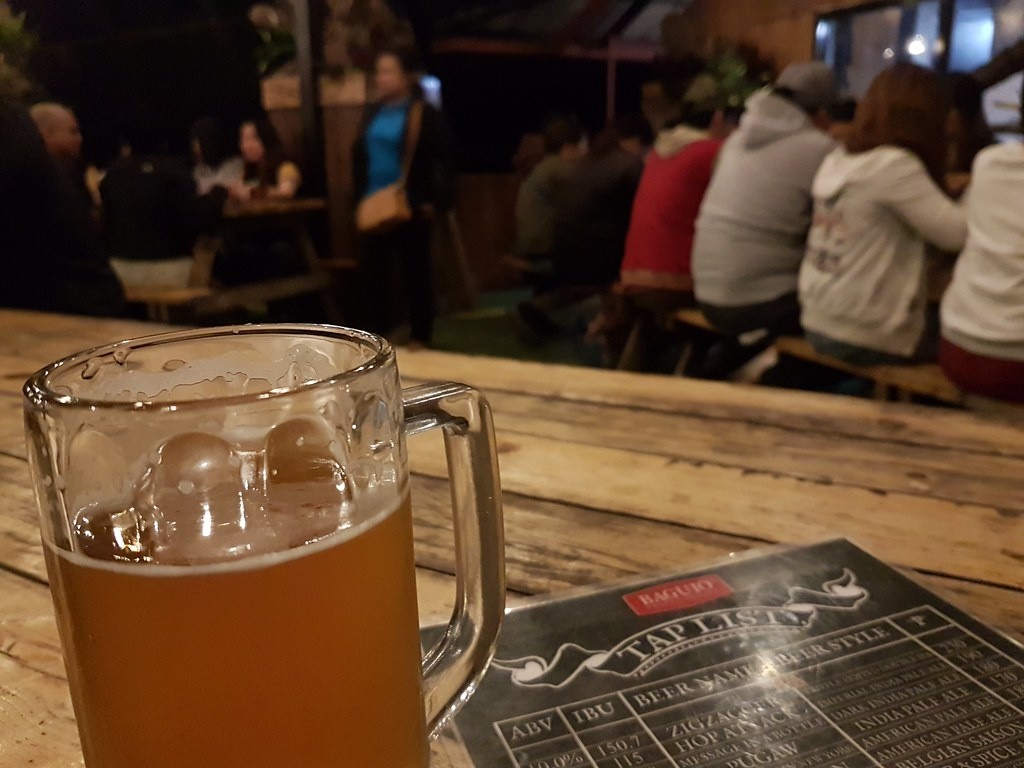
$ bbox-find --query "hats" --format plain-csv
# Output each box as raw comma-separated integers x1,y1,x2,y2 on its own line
776,61,838,112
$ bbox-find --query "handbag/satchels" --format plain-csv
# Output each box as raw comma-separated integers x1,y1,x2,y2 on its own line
356,183,410,232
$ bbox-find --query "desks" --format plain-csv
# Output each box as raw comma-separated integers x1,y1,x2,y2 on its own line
220,200,329,265
0,310,1024,768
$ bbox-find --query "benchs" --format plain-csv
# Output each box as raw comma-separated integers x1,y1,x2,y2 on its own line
615,283,1023,414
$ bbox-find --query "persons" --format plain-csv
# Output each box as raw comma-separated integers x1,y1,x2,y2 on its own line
515,56,1024,401
347,49,450,346
1,98,306,323
